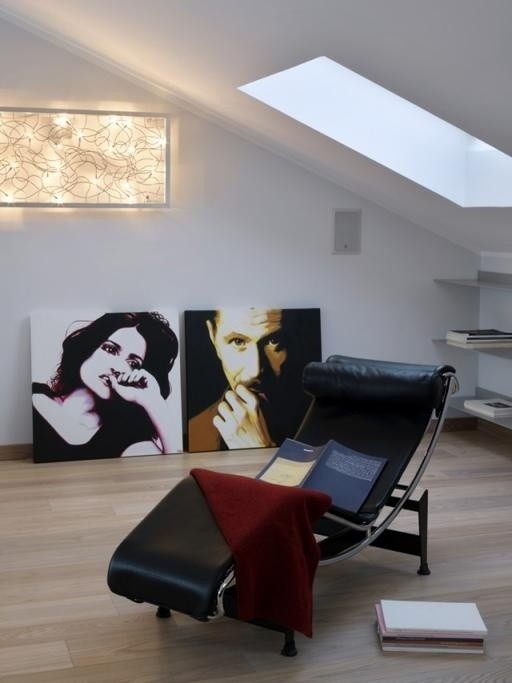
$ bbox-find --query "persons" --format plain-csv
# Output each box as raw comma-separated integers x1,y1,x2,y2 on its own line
187,308,310,450
30,312,184,464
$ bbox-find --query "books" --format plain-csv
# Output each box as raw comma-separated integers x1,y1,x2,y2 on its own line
374,597,487,657
464,395,512,420
445,324,512,346
257,436,390,518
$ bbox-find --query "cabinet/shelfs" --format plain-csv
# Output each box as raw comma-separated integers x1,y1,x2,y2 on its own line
431,279,512,430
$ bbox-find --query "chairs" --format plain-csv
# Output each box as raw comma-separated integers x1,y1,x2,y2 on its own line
108,355,460,657
